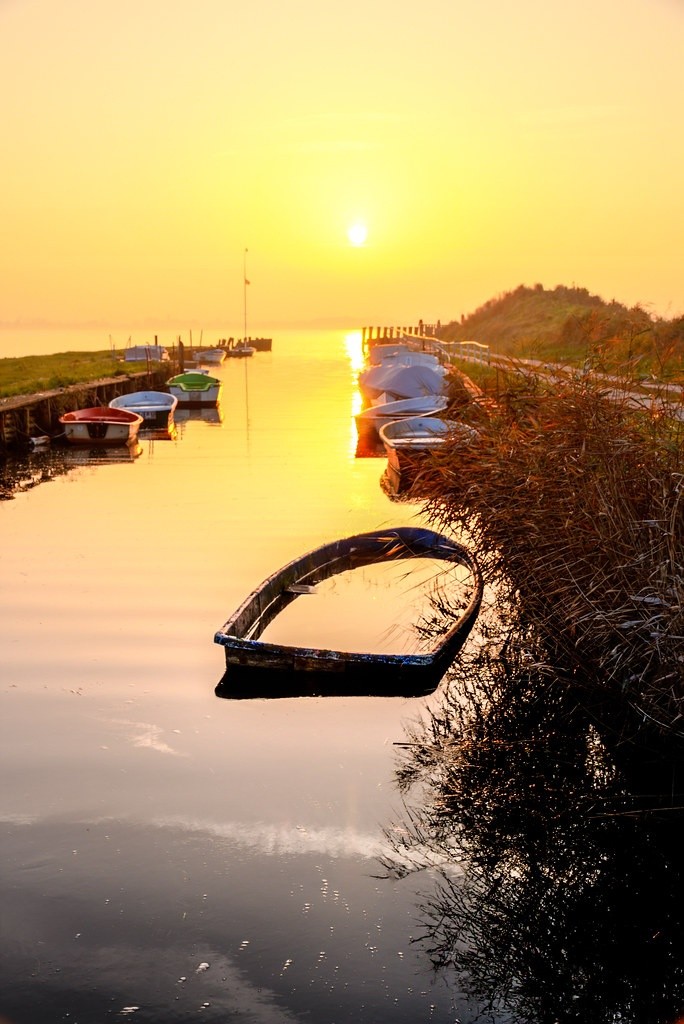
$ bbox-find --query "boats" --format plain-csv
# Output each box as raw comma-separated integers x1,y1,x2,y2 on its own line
352,393,454,432
138,421,183,441
379,414,479,470
165,372,223,405
106,390,179,432
211,526,483,680
196,349,228,364
383,461,418,495
362,338,455,404
173,401,221,423
57,405,144,442
53,443,144,465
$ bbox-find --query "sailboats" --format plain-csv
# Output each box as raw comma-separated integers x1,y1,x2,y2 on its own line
234,247,257,357
